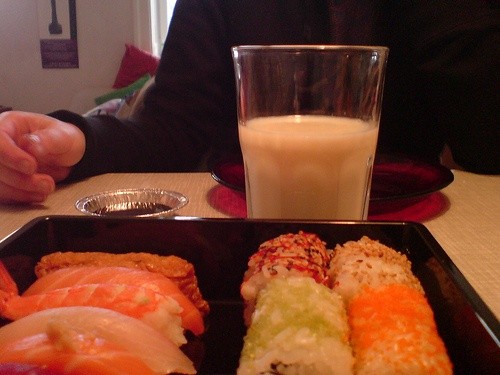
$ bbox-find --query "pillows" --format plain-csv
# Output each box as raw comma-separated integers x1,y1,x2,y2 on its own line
93,42,162,110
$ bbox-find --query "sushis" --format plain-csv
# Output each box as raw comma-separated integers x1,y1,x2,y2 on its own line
233,231,453,375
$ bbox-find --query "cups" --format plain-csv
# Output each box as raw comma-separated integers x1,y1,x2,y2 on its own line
231,44,390,221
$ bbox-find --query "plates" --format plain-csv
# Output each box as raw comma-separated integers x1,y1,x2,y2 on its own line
210,153,456,213
74,188,189,218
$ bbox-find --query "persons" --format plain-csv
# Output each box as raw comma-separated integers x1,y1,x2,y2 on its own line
0,1,500,204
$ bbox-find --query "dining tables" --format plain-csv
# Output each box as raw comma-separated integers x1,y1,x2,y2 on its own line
0,171,500,375
